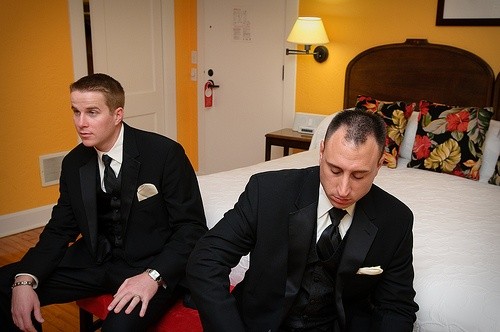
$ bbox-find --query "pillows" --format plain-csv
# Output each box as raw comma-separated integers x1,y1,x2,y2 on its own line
355,94,416,168
407,100,493,181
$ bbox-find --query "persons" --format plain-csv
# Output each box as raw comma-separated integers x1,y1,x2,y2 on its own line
189,107,420,332
0,71,212,332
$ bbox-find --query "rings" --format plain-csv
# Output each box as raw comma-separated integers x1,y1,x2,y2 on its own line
134,295,141,300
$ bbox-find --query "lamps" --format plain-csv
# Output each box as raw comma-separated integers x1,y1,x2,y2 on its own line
285,16,329,63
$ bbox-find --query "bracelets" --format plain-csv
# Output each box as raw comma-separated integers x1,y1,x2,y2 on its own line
10,281,34,288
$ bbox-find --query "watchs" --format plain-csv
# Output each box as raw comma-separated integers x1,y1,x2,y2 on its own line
143,267,163,285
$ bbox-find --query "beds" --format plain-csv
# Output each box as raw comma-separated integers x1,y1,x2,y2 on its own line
76,39,500,332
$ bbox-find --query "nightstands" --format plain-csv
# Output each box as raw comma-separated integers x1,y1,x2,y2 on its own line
265,128,313,162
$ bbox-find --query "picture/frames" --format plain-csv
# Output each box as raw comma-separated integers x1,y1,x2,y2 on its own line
436,0,500,27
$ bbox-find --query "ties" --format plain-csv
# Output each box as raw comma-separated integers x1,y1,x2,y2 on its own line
316,206,348,261
102,155,116,195
